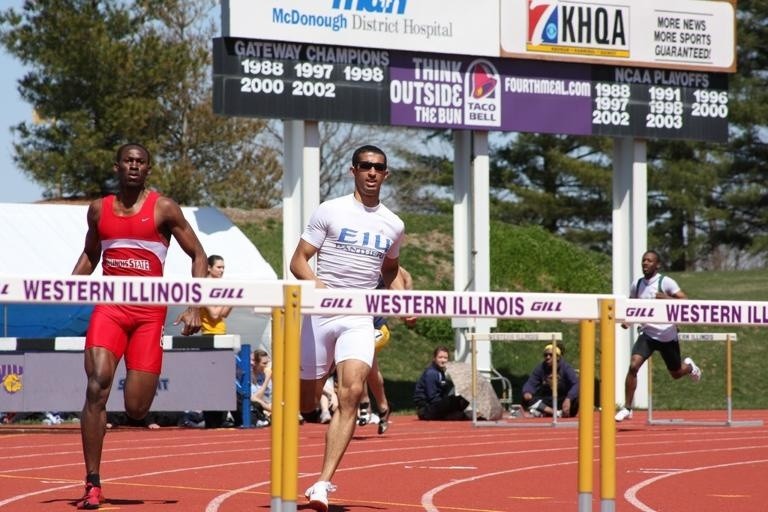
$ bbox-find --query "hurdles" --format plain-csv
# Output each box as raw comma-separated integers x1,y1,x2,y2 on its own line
561,298,768,512
253,288,626,512
640,332,763,426
0,275,315,512
466,332,579,429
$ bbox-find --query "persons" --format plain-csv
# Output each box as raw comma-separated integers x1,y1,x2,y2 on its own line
290,146,417,511
200,255,233,334
71,144,208,509
359,266,417,434
414,346,472,420
614,251,700,422
522,344,578,417
251,350,380,427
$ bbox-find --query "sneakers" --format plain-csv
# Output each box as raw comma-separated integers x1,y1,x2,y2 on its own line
615,408,632,422
304,480,337,512
378,406,391,434
557,410,563,419
77,482,104,509
684,357,701,383
530,409,544,418
320,410,331,424
359,402,371,426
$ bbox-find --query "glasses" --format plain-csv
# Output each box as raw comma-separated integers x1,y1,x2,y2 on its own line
543,352,553,357
353,160,387,172
